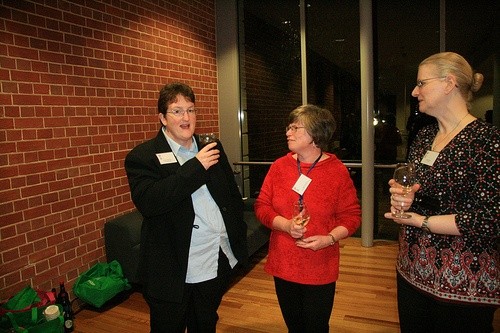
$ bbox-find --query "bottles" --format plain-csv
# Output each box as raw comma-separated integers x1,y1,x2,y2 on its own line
234,171,242,196
57,280,69,305
62,293,73,333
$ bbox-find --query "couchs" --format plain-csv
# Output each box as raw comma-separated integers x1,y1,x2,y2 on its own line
104,199,271,287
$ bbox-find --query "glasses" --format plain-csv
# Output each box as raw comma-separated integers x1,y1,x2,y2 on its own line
417,77,459,88
286,124,306,131
166,107,199,117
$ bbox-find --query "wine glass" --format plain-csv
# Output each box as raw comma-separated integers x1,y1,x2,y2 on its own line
393,164,416,219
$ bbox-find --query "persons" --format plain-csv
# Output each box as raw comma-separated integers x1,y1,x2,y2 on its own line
253,104,363,333
384,52,500,333
125,82,248,333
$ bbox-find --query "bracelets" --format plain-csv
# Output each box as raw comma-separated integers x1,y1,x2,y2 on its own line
328,234,336,244
420,215,430,231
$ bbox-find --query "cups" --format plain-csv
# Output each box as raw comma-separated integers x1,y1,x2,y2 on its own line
294,203,310,228
199,133,216,150
44,305,59,321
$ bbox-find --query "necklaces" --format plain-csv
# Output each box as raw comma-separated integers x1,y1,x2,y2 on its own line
432,112,469,150
